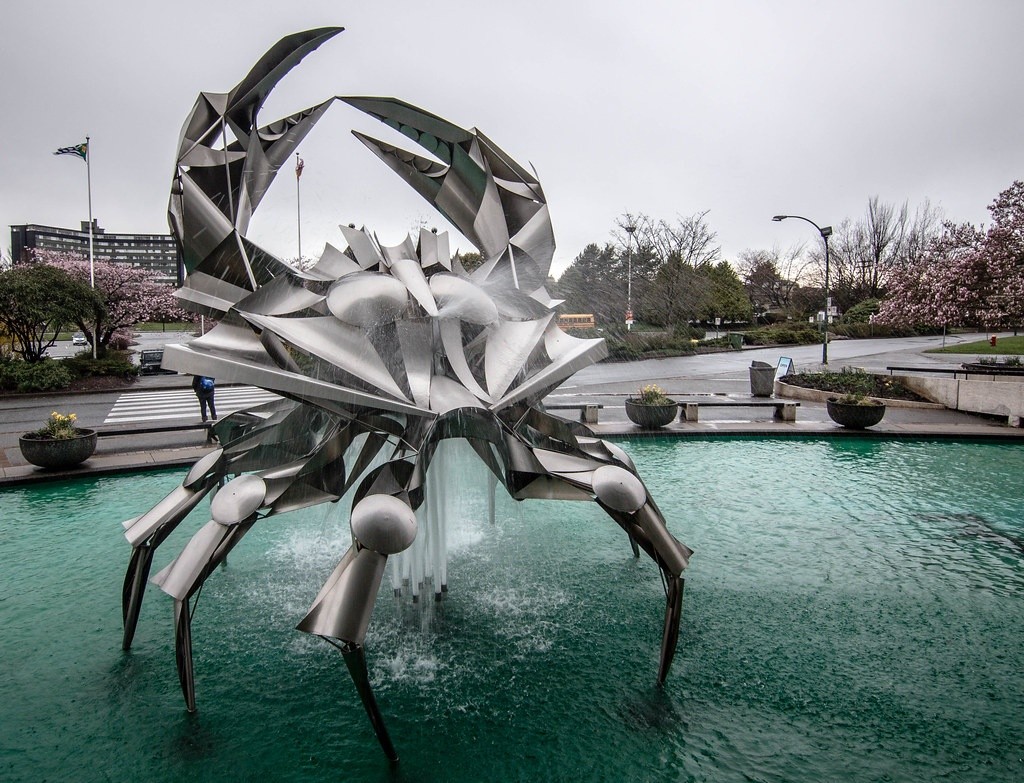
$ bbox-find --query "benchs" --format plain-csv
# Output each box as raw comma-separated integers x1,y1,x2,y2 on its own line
83,421,216,455
544,403,604,424
677,401,801,423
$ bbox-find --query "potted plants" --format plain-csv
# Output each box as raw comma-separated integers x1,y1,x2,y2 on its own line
625,384,678,428
18,412,97,469
826,390,887,429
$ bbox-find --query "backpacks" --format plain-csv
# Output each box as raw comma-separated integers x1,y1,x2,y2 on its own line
200,376,215,393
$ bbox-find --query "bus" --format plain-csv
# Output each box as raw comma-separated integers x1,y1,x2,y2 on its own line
556,314,595,332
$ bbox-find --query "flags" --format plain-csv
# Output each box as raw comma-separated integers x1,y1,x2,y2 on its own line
294,158,304,177
52,142,87,162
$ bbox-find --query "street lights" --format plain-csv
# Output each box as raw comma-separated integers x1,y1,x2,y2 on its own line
625,226,636,331
772,215,830,364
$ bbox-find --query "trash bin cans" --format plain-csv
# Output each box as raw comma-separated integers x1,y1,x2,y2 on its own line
749,360,776,398
729,332,743,345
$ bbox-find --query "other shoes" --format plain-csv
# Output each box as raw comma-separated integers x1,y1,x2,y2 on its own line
202,416,208,422
212,415,217,420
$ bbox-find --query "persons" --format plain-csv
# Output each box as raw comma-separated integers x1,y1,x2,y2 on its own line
190,374,220,424
